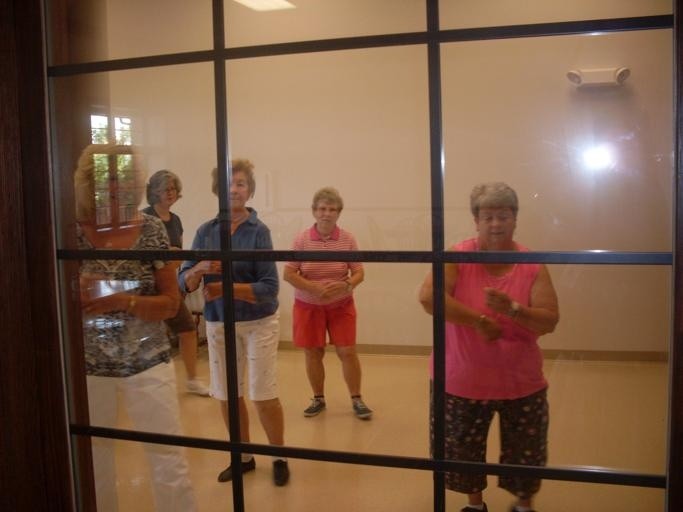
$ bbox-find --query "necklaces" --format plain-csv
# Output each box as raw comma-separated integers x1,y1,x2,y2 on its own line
318,231,331,236
99,235,112,247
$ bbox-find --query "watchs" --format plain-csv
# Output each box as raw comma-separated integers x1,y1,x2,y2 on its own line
510,301,520,322
345,280,353,292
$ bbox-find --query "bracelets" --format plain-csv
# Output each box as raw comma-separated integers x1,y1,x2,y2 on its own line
127,296,135,313
475,315,486,331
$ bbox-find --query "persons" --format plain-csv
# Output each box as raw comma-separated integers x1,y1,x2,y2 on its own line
75,144,196,512
285,188,375,418
179,159,290,486
141,168,211,397
418,183,558,512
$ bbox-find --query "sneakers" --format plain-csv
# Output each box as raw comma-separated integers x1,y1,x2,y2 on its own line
353,400,374,418
186,378,211,396
218,457,256,483
460,501,488,512
272,459,289,486
303,400,326,417
